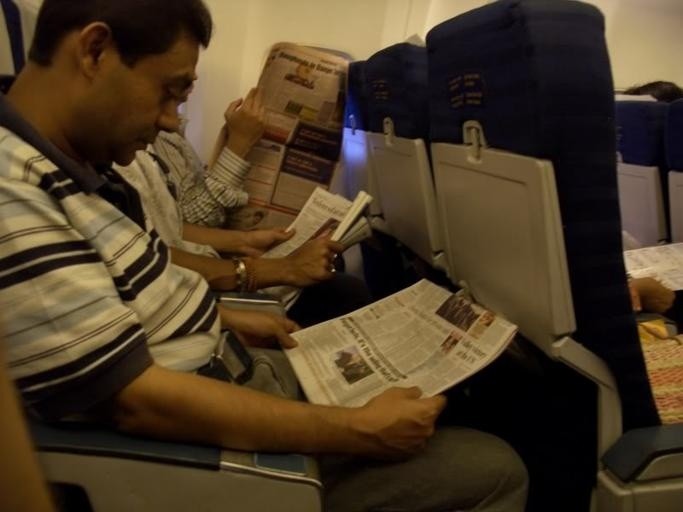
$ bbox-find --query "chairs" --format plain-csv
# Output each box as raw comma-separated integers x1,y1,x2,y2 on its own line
358,42,447,275
615,94,665,245
339,59,387,231
425,0,682,512
0,0,326,512
665,97,682,247
5,0,39,77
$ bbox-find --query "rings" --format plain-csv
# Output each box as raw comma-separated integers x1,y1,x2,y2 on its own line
334,251,342,262
328,263,338,274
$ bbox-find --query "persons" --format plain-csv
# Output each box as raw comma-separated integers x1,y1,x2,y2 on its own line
153,84,271,228
632,275,682,334
241,211,264,228
0,0,530,512
105,147,373,324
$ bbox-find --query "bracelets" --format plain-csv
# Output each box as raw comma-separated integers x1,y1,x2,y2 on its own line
242,255,257,294
233,255,246,294
